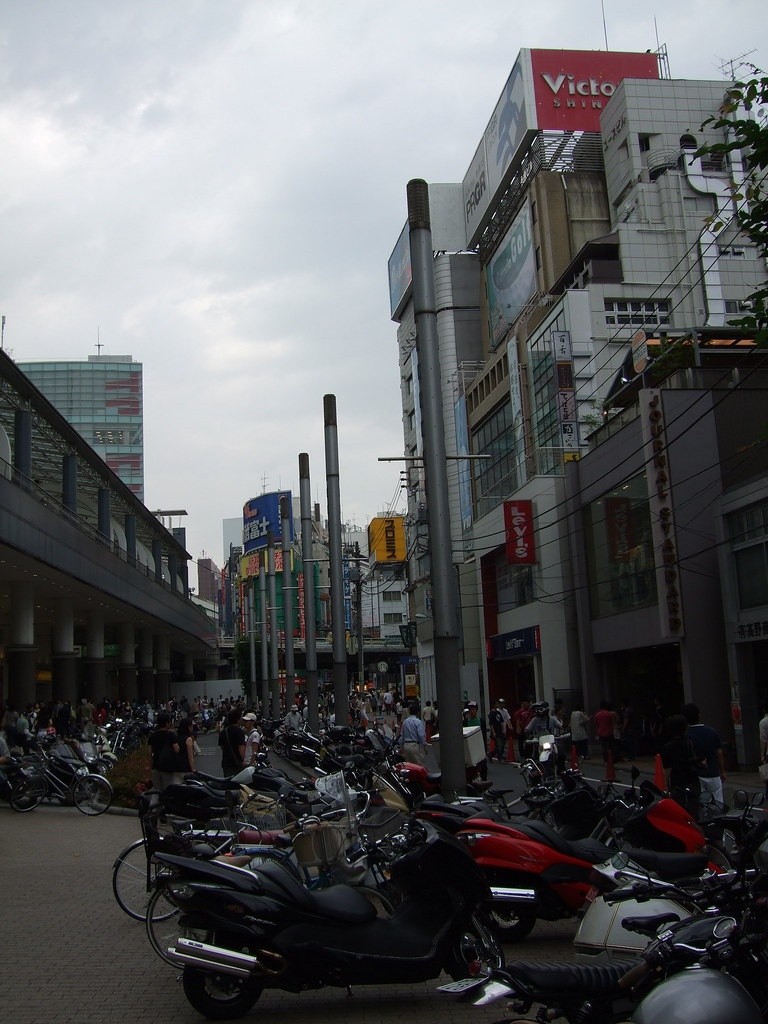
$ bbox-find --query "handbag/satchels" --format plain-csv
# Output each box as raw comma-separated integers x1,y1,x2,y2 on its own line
430,712,435,723
255,748,270,765
232,761,245,774
758,763,768,783
149,729,171,757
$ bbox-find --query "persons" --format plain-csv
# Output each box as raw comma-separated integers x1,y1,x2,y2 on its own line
759,702,768,798
348,679,440,767
269,685,336,737
0,695,263,792
657,703,727,819
462,695,662,781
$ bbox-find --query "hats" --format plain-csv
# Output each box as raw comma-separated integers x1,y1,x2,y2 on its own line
468,701,478,708
290,704,298,711
241,712,257,721
462,709,470,714
498,699,505,703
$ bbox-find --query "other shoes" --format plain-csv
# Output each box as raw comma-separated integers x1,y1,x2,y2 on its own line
487,754,493,764
498,759,506,764
584,756,591,759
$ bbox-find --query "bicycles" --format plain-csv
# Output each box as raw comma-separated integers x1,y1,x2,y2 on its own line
9,742,115,818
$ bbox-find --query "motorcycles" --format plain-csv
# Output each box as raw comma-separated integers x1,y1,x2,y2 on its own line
19,706,160,806
167,702,228,735
257,716,438,806
143,722,768,1023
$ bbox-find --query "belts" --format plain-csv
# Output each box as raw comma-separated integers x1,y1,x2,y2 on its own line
404,740,418,744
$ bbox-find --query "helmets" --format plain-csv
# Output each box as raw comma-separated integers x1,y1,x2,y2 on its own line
534,701,549,717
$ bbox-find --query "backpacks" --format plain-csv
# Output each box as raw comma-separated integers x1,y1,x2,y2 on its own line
166,735,189,772
